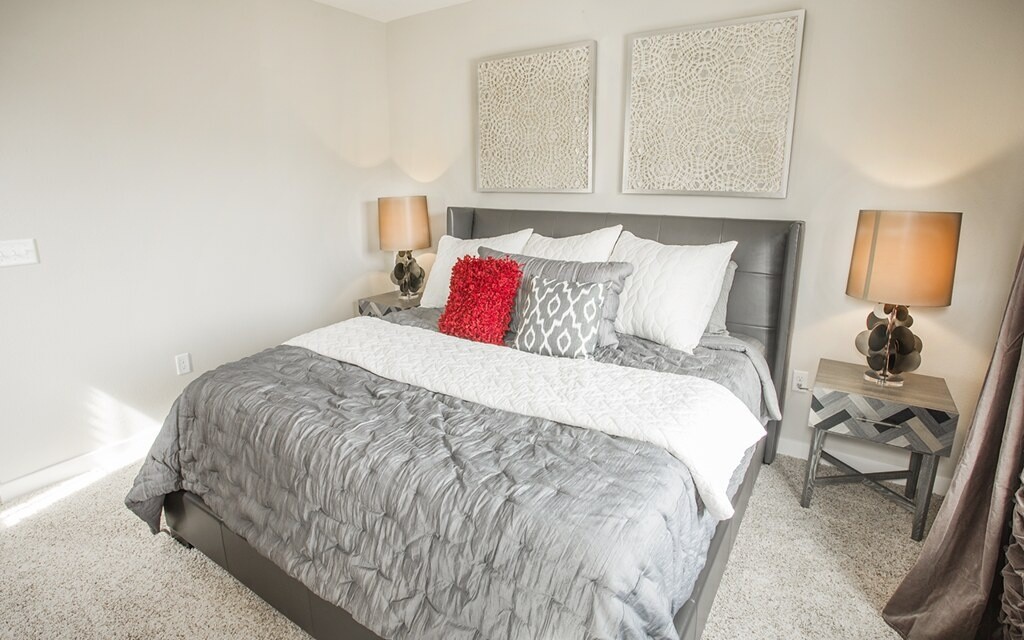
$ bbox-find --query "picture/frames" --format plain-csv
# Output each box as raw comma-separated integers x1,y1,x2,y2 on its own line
475,38,598,194
620,7,806,199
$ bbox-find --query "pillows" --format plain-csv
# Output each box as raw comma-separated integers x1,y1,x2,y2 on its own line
477,244,634,350
522,222,623,263
512,275,614,360
607,231,739,355
420,227,534,309
439,254,526,346
704,260,738,338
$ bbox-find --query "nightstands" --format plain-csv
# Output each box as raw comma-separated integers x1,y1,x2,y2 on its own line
800,357,960,542
358,289,424,317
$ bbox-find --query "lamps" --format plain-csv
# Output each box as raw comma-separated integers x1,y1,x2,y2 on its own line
846,209,962,387
376,195,431,300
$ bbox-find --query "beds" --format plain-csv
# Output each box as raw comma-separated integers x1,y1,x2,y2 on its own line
124,206,808,640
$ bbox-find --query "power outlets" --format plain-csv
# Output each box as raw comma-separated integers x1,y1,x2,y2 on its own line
792,370,809,392
176,352,191,375
0,238,40,266
